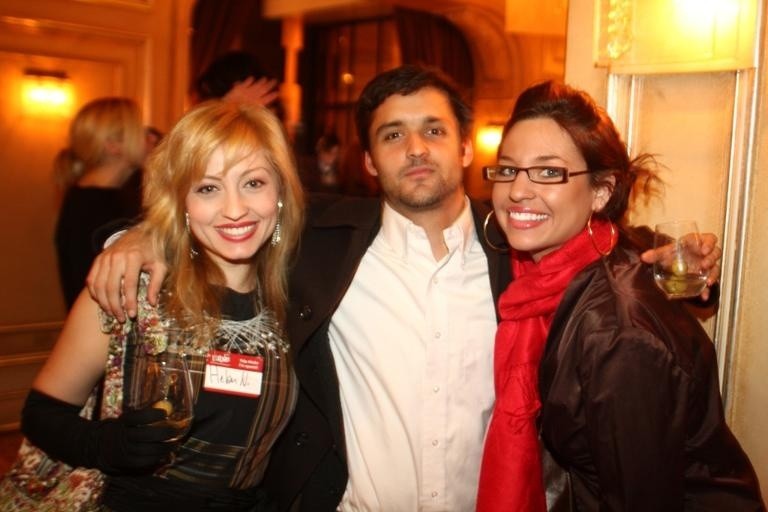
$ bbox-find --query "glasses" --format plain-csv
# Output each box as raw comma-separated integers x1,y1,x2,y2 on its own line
482,165,596,184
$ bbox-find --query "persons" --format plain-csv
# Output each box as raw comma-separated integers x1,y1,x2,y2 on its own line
144,125,165,158
0,96,310,512
475,80,767,512
87,61,721,512
318,127,341,189
55,76,285,311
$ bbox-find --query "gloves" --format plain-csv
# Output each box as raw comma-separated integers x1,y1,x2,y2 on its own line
20,388,179,478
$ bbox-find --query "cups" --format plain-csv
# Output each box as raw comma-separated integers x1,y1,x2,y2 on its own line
128,329,197,447
653,221,711,301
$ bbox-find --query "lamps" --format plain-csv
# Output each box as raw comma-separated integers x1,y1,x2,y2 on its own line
477,119,509,151
21,67,75,117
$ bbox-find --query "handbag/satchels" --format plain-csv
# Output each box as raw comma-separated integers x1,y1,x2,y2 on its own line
2,436,108,511
